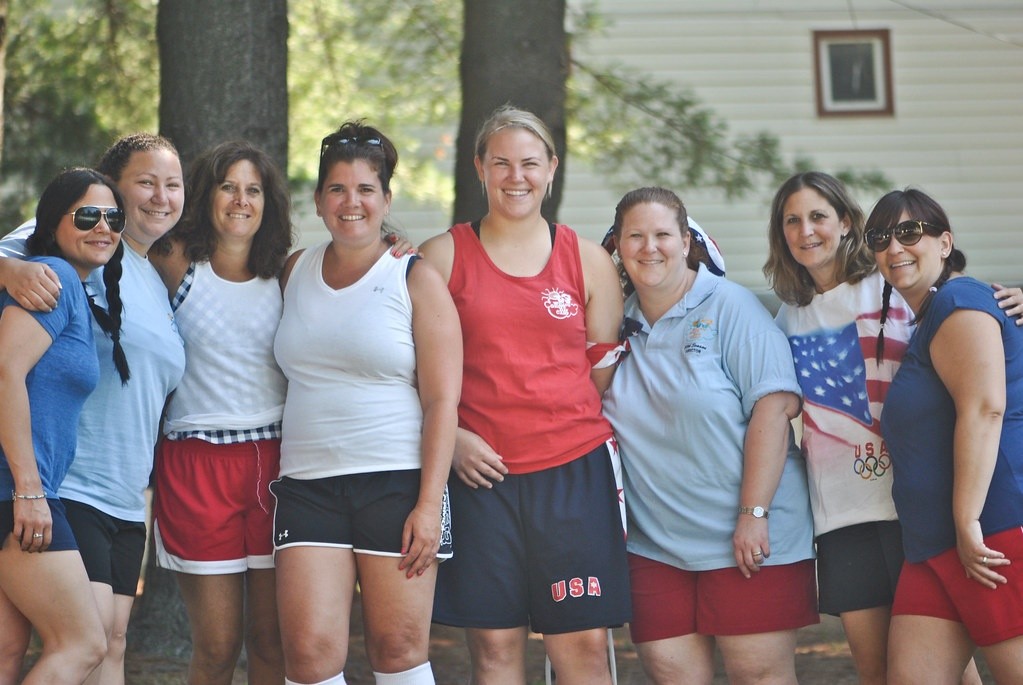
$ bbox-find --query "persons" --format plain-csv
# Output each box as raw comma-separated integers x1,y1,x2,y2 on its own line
1,131,186,685
760,172,1023,685
0,168,131,685
601,187,822,685
862,184,1023,685
273,116,465,685
148,138,428,685
415,103,645,685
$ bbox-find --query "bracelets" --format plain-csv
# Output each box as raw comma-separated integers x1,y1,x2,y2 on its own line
11,489,48,502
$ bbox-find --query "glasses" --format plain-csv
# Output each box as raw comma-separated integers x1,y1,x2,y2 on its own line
320,133,384,161
864,219,942,251
64,205,127,233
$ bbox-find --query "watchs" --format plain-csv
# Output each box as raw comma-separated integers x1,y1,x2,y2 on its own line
739,506,771,521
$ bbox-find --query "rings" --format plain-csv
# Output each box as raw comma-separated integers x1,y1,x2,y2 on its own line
983,556,988,567
33,533,43,538
753,551,762,556
